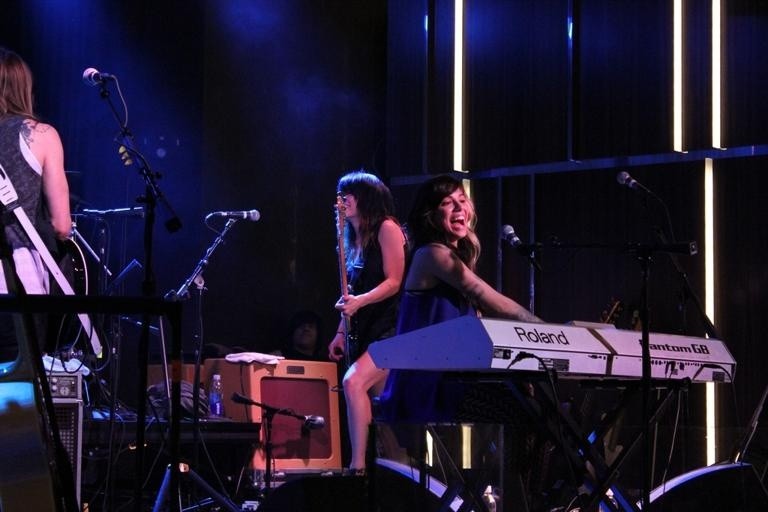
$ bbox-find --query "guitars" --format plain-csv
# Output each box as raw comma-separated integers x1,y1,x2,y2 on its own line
334,193,360,366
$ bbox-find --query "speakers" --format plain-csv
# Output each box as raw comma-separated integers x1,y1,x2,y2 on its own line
40,371,83,510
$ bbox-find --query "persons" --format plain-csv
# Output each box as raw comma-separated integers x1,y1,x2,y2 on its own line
275,311,327,361
384,170,551,451
1,47,74,380
327,168,410,476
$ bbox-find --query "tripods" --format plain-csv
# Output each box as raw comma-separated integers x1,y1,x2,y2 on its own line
151,313,239,512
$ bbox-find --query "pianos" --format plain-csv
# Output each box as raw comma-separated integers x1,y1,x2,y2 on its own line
366,317,737,512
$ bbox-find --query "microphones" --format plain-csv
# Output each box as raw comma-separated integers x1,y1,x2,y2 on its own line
82,66,114,89
214,207,260,223
617,169,664,205
305,414,326,429
84,203,145,219
501,223,546,275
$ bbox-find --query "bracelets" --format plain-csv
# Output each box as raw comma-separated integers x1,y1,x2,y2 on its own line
334,330,346,336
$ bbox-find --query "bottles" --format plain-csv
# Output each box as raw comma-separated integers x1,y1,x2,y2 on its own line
483,484,497,512
208,374,224,419
